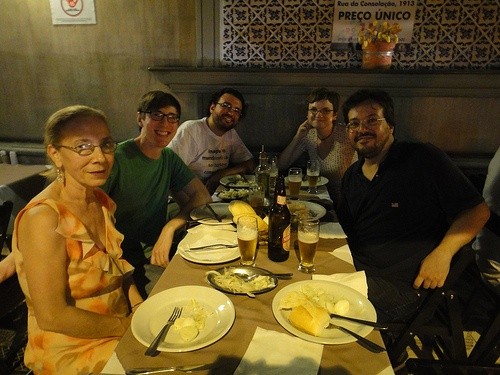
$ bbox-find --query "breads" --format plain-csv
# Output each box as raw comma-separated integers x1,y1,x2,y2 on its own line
288,302,331,337
228,200,269,231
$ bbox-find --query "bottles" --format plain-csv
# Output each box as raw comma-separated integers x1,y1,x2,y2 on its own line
268,175,290,261
255,152,269,199
267,156,279,192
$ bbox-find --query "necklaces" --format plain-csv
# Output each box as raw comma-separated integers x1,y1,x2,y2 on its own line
317,122,334,141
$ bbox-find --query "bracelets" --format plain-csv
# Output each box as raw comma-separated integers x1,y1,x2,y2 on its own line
129,300,143,307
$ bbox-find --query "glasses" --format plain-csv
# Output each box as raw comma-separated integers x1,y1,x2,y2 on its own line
56,141,118,156
307,109,335,114
346,118,387,133
139,111,179,123
215,103,243,115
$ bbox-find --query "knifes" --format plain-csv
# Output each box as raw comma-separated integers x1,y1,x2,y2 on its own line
325,322,387,354
205,203,222,222
125,362,222,375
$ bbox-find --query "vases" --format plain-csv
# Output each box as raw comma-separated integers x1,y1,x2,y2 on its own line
362,41,397,69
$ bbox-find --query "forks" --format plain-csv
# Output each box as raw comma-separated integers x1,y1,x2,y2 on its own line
144,306,182,356
189,244,238,250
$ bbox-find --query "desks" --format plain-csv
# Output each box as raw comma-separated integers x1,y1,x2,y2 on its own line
101,184,396,375
0,164,54,188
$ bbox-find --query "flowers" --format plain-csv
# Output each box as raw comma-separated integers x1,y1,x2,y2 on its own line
356,17,403,48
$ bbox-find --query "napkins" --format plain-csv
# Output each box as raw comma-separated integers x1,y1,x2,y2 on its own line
186,223,238,233
319,221,349,238
233,326,324,375
312,270,369,298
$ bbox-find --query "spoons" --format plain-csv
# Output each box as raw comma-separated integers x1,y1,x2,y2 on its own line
234,272,293,279
186,213,227,223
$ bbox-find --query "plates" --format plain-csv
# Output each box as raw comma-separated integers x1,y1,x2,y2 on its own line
206,264,278,294
286,199,326,223
216,188,249,200
272,279,377,344
177,229,242,264
130,284,236,353
190,202,234,224
284,175,329,188
220,174,255,187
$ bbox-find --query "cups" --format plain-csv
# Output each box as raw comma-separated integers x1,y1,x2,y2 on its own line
237,216,259,267
307,160,319,194
288,168,303,201
249,182,266,216
298,216,320,273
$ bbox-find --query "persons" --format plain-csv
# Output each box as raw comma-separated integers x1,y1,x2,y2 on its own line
0,91,211,296
12,106,144,375
278,88,358,201
337,88,490,371
472,147,500,270
166,88,254,220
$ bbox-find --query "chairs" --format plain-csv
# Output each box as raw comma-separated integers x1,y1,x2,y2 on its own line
0,200,36,375
388,205,500,375
